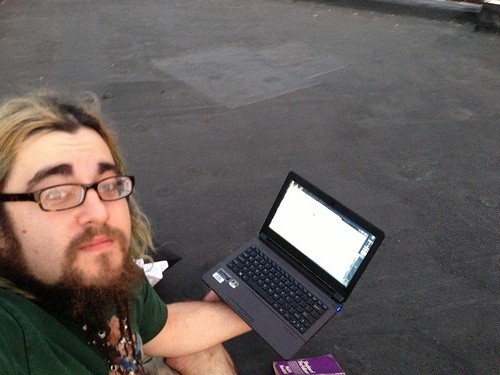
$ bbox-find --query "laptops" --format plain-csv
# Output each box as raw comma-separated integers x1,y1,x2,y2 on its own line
201,172,386,361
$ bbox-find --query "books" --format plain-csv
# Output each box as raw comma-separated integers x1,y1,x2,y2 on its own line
271,353,346,375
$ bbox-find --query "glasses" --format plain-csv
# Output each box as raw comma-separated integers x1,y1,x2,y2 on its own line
1,174,135,213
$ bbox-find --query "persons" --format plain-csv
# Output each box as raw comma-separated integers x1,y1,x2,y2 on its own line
0,88,256,375
